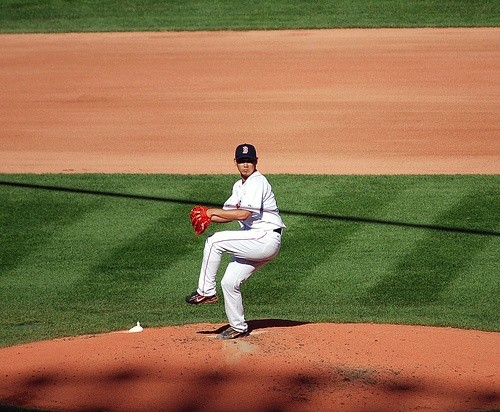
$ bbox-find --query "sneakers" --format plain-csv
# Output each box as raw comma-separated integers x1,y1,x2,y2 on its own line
221,327,249,339
186,291,218,305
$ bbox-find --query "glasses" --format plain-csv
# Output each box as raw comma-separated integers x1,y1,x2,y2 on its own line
237,159,254,163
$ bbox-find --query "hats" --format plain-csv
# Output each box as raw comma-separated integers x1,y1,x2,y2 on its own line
236,144,256,161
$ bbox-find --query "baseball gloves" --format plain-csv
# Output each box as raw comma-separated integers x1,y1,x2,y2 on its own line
190,205,212,235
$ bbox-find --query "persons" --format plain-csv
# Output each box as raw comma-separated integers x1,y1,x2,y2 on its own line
185,144,286,339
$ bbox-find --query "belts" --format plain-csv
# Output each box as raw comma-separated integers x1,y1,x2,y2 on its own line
274,228,281,234
